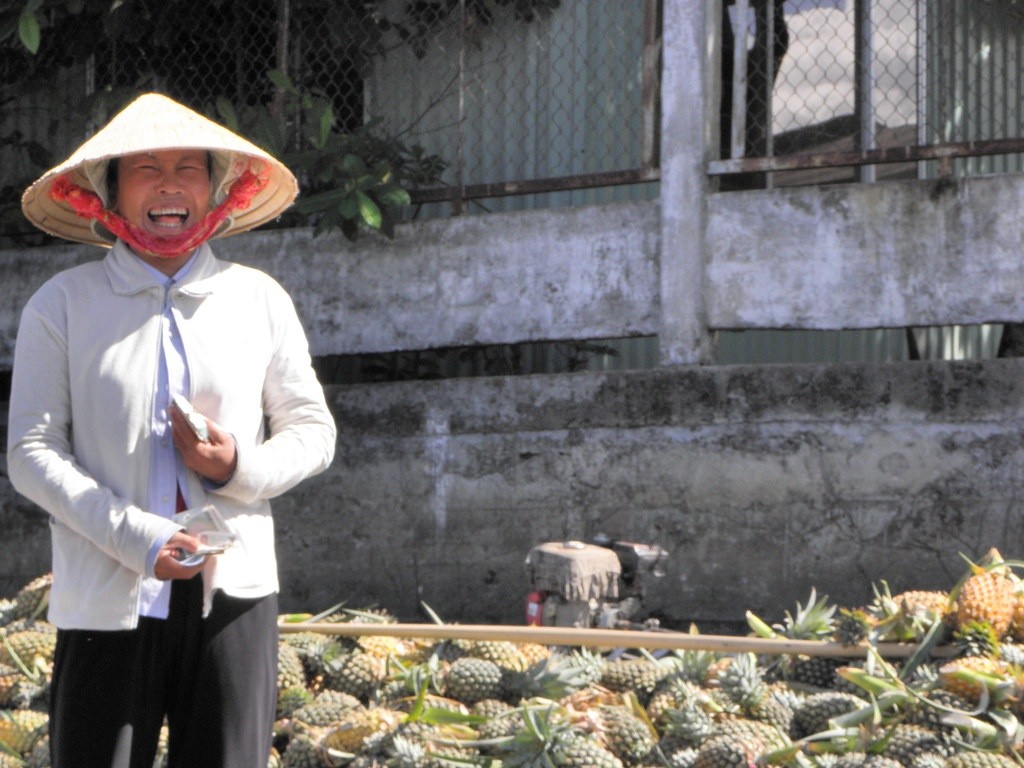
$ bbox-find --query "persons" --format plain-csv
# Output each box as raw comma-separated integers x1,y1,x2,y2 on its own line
5,93,337,768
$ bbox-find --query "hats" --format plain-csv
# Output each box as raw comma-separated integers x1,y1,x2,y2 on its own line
20,93,299,257
85,149,233,245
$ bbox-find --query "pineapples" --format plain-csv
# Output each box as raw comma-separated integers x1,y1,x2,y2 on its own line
265,546,1024,768
0,572,169,768
